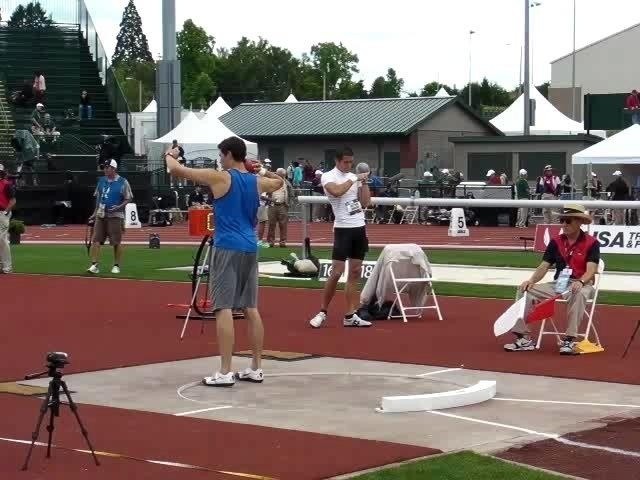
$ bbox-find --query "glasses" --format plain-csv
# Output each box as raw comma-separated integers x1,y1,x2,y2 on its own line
560,218,572,224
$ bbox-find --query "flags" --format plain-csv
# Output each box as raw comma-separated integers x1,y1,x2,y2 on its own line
527,293,563,326
493,295,528,338
569,337,605,355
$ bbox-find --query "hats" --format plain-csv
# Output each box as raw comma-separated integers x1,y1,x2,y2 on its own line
101,159,117,169
424,172,433,177
519,169,527,175
612,171,622,176
441,169,449,174
554,204,592,224
544,164,552,170
486,170,496,176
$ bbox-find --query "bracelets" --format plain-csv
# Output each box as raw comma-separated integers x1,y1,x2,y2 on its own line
165,152,175,157
577,279,585,286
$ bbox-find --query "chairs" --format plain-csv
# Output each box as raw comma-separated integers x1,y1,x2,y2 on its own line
386,243,443,323
535,258,605,354
388,188,422,225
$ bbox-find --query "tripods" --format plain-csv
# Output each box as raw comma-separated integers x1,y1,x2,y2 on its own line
180,240,213,340
22,366,101,471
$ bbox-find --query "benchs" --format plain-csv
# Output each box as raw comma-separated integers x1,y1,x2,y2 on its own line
0,28,134,170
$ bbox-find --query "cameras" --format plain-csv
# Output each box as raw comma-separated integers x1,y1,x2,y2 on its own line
88,217,95,227
47,351,68,363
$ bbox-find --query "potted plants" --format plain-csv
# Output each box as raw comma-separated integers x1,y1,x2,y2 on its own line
8,219,25,245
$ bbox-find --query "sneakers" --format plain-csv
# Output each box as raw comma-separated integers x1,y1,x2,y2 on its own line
504,338,535,351
112,266,120,274
257,240,286,248
560,340,573,353
236,368,264,383
202,370,235,386
310,311,327,327
344,314,372,326
88,265,99,273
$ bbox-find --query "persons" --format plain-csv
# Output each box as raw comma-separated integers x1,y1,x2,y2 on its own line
309,148,373,329
256,158,335,249
163,136,284,386
31,103,46,133
32,70,47,101
78,89,98,122
626,89,640,125
0,163,16,274
40,112,60,144
170,139,186,190
605,169,630,224
365,165,573,229
502,201,601,355
585,172,602,217
86,158,131,274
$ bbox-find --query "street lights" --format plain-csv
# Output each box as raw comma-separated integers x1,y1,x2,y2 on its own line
506,42,523,96
321,61,331,102
467,27,476,108
524,0,541,136
